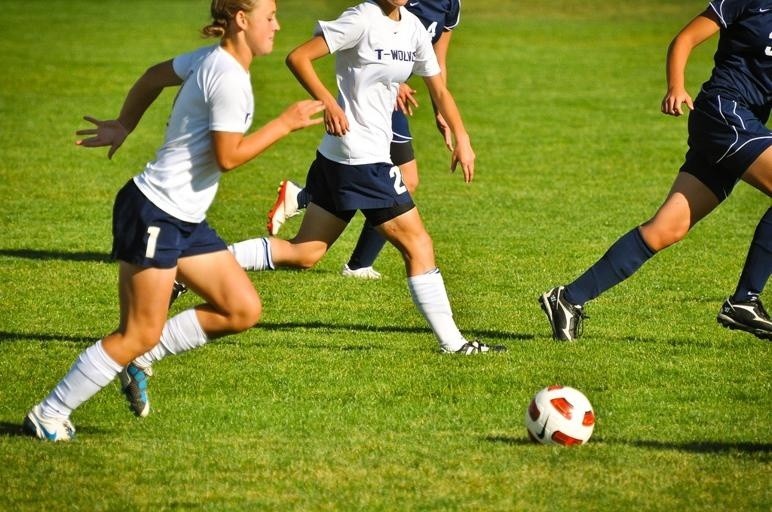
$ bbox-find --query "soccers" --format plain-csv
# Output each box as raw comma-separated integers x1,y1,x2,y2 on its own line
525,384,595,444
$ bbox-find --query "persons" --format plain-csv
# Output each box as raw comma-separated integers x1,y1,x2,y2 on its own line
538,0,772,344
22,0,325,442
265,0,460,277
168,0,506,357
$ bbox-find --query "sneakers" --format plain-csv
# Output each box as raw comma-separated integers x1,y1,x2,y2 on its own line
717,294,770,343
263,177,302,237
439,339,508,362
169,280,189,311
537,288,588,342
19,406,76,444
339,262,390,284
120,363,156,418
